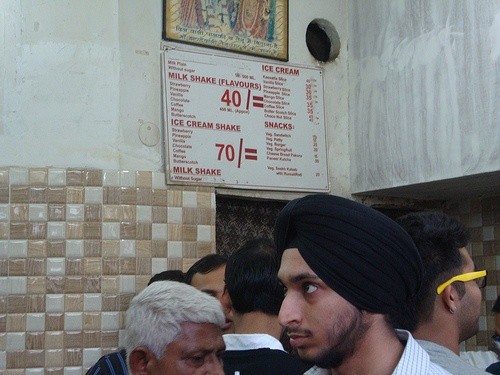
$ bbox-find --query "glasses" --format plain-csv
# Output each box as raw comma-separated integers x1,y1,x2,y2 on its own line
434,267,489,294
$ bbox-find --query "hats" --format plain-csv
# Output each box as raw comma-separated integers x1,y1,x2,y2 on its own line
274,194,421,314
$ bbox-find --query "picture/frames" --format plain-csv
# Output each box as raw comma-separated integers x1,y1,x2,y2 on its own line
163,0,290,62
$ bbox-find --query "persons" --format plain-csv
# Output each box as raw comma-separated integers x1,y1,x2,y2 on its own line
185,255,236,335
484,294,500,375
272,194,453,375
124,281,226,375
394,212,495,375
222,239,308,374
85,270,187,375
